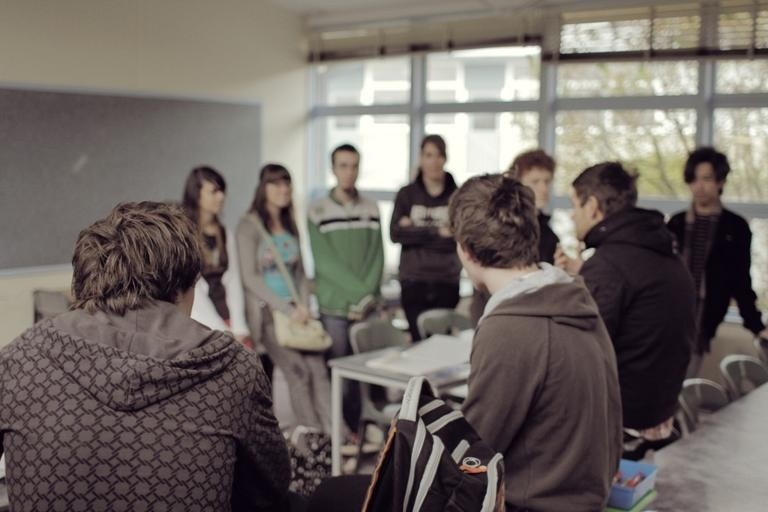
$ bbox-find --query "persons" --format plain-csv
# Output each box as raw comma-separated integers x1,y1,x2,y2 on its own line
0,200,290,512
308,144,384,445
310,174,624,512
666,147,768,352
390,136,460,397
470,151,560,328
553,162,696,442
235,164,351,442
184,167,254,350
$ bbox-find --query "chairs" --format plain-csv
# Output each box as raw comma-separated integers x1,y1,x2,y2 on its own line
720,354,768,399
348,319,407,473
416,308,475,339
753,336,767,370
678,378,731,430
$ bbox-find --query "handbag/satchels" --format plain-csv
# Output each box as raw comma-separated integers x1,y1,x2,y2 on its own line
284,426,332,492
271,305,332,352
361,373,508,512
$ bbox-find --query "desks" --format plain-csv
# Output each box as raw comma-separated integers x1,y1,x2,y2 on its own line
326,342,471,476
622,383,768,512
